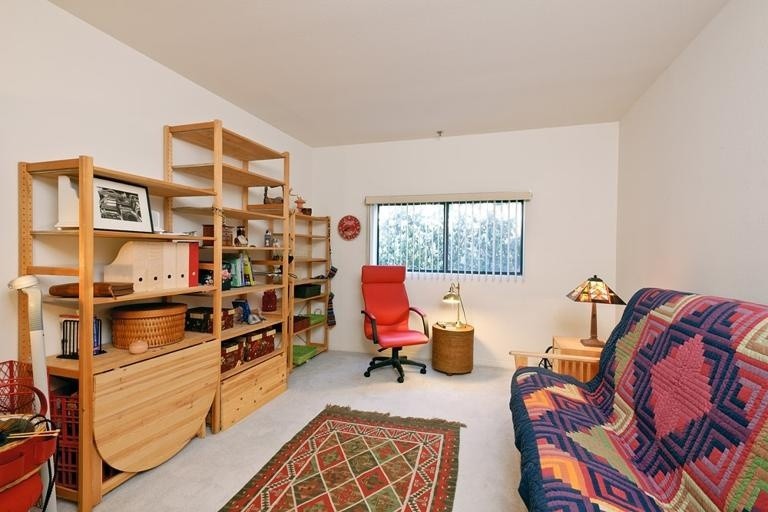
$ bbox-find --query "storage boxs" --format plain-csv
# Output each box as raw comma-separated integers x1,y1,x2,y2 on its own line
110,302,276,373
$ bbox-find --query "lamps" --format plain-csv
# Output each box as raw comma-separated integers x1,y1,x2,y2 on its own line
442,282,465,328
566,275,626,347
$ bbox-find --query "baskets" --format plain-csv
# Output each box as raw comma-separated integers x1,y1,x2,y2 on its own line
110,303,188,349
49,390,79,491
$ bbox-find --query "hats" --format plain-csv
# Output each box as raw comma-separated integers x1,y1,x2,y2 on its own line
337,215,361,241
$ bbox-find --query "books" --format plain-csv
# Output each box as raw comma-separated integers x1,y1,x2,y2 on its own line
49,283,133,297
222,250,256,287
232,299,250,322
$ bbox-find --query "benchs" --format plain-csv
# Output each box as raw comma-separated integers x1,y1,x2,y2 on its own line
509,288,768,512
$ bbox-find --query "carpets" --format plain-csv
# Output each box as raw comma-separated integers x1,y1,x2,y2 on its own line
218,406,467,512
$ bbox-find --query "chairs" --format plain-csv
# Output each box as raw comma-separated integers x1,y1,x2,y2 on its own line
361,265,429,383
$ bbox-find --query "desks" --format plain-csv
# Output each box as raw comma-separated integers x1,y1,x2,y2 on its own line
553,336,603,384
432,324,474,376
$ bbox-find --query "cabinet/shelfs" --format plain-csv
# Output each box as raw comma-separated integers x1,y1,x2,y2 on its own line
18,155,222,512
164,119,289,434
248,205,331,373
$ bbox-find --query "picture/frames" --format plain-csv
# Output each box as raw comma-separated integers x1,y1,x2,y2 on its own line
93,175,155,233
